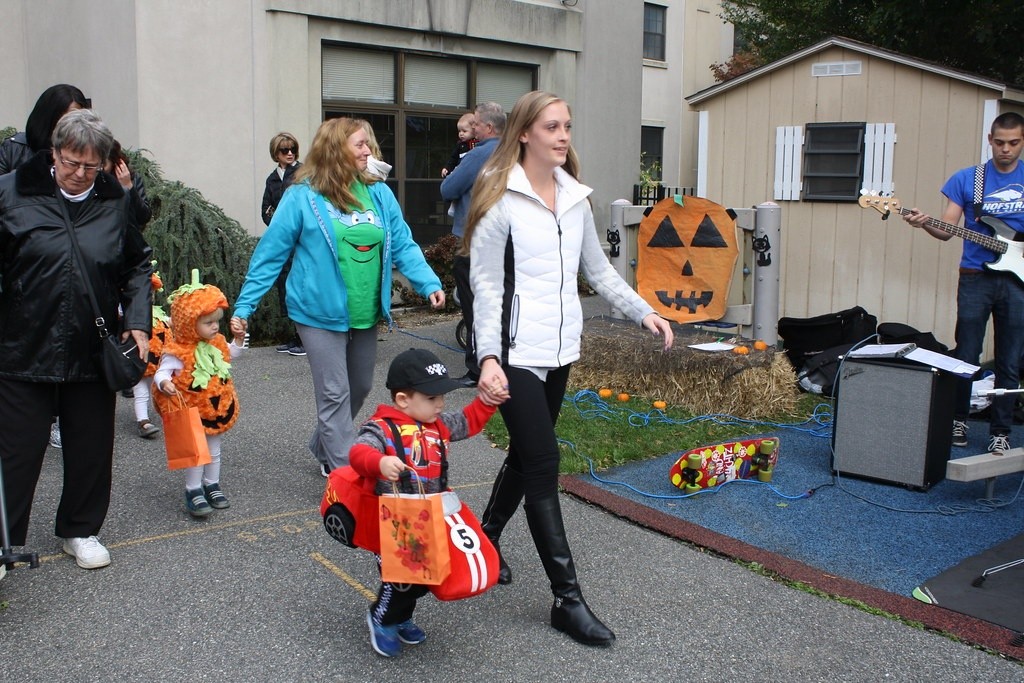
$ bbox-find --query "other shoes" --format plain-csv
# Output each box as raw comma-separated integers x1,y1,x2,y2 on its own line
448,202,455,218
137,419,158,438
121,387,134,398
321,463,330,477
48,424,61,448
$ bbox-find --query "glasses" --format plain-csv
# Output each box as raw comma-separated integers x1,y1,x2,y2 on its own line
59,148,104,174
276,147,297,155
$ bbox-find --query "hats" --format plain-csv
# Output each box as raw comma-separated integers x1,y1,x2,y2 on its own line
386,346,465,397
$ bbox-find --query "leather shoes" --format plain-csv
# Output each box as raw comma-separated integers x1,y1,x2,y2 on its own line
451,369,481,389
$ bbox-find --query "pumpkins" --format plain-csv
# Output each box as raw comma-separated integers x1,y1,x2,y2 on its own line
733,346,749,354
598,388,612,398
754,340,767,350
618,392,629,401
653,399,666,409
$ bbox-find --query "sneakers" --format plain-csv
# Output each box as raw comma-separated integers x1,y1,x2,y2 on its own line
203,482,229,509
953,418,968,447
395,618,426,645
275,341,302,354
287,344,307,356
0,549,7,579
987,433,1012,451
366,610,400,657
184,487,213,517
62,533,111,569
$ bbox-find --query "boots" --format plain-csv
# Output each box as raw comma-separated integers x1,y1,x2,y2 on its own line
481,464,525,585
525,493,615,648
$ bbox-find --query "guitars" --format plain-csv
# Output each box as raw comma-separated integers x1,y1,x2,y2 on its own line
856,186,1024,283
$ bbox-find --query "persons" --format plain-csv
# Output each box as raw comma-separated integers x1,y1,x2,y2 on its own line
349,349,510,659
441,113,480,218
232,117,448,477
152,269,250,517
903,112,1024,453
440,101,507,388
354,119,392,181
0,84,173,448
0,109,152,582
261,131,307,355
456,93,674,648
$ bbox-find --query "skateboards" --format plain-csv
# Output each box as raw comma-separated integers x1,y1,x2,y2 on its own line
670,437,781,494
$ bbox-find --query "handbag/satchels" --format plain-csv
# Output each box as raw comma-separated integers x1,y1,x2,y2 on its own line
452,222,515,383
779,305,948,394
377,465,451,585
100,316,148,392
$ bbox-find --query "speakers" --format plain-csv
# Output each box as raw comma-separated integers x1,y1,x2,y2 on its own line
829,354,955,492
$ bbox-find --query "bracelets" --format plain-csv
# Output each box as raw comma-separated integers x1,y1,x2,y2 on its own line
481,355,497,361
